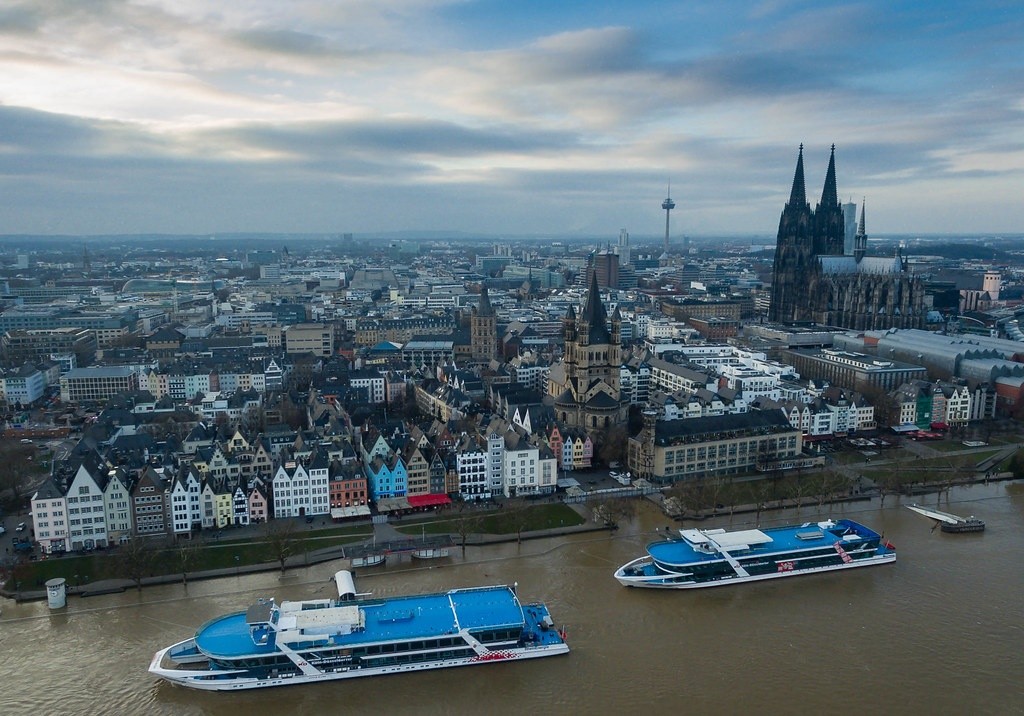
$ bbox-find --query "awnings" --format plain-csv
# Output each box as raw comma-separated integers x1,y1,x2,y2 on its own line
407,493,452,507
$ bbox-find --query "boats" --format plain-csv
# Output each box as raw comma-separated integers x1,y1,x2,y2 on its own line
613,516,898,590
941,515,985,532
350,555,386,568
148,581,570,692
412,547,448,558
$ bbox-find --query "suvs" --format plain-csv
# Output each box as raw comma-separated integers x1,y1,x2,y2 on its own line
16,523,25,531
610,472,618,479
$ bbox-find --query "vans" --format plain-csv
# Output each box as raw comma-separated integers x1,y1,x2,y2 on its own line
21,439,33,444
